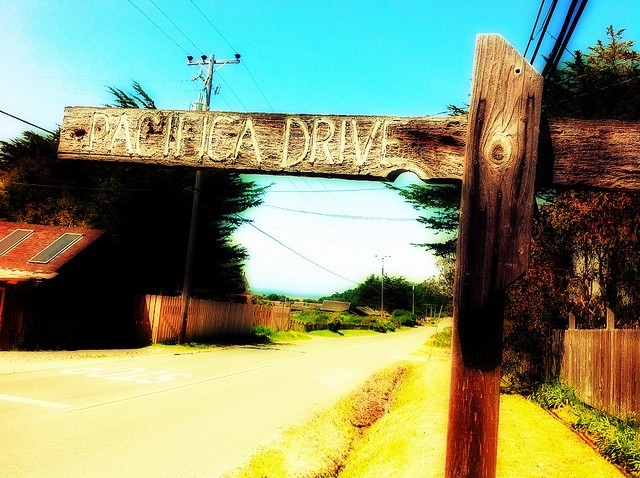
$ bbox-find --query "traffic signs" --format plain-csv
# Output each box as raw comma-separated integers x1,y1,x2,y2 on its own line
56,103,468,185
